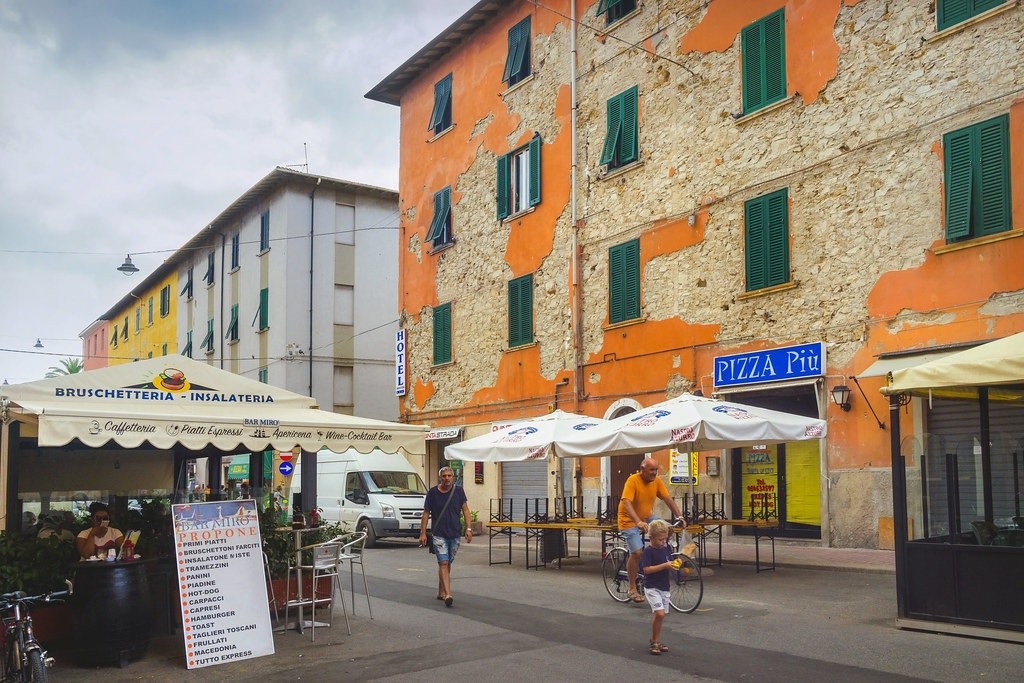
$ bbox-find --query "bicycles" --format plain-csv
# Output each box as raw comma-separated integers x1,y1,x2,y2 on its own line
602,518,704,614
0,578,73,683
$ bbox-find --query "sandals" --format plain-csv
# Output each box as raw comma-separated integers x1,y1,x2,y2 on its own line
650,642,661,654
649,638,669,652
627,592,644,603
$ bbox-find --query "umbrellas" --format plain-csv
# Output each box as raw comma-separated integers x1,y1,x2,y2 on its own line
557,393,827,516
445,409,610,516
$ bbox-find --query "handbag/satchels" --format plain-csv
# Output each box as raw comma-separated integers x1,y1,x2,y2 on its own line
428,533,435,554
678,527,696,562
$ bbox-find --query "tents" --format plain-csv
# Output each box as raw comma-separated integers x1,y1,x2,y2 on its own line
884,333,1024,540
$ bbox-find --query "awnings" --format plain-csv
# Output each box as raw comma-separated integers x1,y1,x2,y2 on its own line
228,450,271,480
11,400,430,456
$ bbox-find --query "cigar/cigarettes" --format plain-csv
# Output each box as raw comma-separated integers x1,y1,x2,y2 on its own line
419,544,422,548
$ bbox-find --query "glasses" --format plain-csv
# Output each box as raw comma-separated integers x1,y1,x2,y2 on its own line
94,515,109,522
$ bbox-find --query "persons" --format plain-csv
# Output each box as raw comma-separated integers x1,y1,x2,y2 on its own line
77,503,125,559
236,481,251,499
274,486,286,504
419,467,473,606
24,510,75,541
618,459,686,603
641,520,682,654
195,485,200,493
221,485,224,492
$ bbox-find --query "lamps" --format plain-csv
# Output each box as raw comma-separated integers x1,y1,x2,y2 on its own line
830,380,850,413
117,253,140,277
33,338,45,351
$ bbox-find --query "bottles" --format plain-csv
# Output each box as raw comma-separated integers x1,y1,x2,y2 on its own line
123,540,134,561
309,508,319,527
281,500,288,525
221,482,253,500
179,479,210,503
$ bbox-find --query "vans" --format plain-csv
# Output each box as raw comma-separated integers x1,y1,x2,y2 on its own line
286,449,432,550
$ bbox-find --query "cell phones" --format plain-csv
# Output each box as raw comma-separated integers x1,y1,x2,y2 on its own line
101,521,110,527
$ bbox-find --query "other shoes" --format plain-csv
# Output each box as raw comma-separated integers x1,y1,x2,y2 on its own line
437,595,445,600
445,596,453,607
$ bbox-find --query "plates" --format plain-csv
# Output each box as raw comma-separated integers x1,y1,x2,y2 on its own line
86,558,102,562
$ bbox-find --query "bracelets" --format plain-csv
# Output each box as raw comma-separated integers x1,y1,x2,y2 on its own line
679,516,683,519
466,528,471,530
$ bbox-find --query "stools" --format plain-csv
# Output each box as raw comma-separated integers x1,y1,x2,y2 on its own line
261,532,372,642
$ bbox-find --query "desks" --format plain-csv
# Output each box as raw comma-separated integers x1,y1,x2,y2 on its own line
272,525,331,633
488,492,784,581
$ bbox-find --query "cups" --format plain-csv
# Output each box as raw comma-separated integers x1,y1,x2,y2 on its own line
98,550,107,558
292,514,306,528
672,553,682,570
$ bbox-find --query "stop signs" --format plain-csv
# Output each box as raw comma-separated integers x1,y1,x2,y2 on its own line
279,450,294,461
